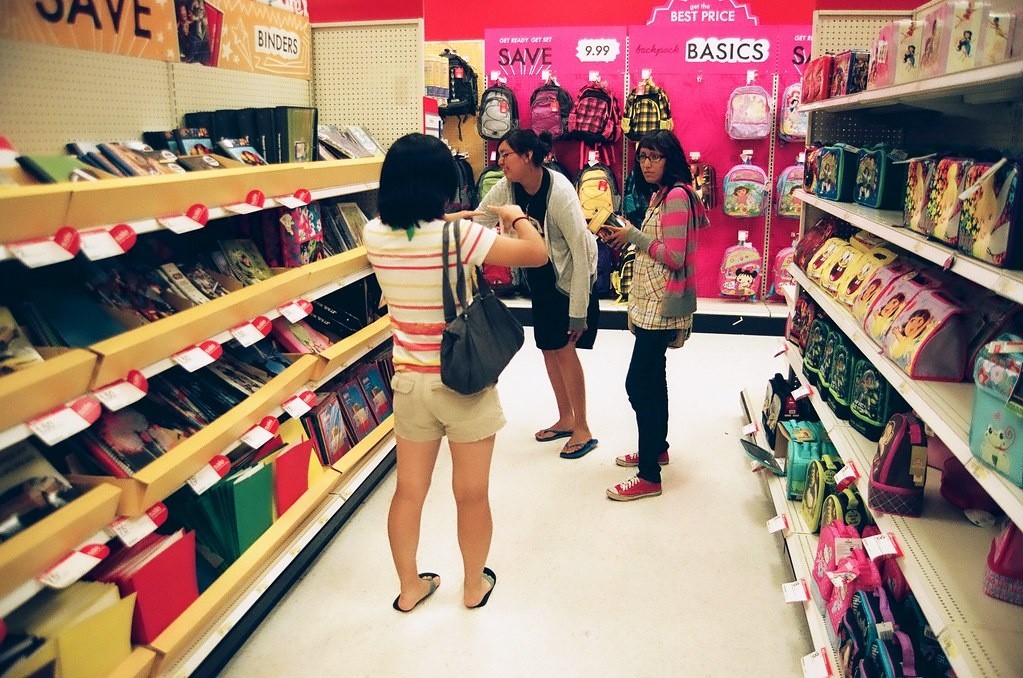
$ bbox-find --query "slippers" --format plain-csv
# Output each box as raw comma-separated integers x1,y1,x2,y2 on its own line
560,438,599,459
393,573,440,612
467,567,496,608
536,429,574,441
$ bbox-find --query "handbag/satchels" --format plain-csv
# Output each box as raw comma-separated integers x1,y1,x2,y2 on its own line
440,221,525,395
628,311,692,348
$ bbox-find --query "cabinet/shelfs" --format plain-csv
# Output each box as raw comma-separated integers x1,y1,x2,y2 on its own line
740,59,1023,678
0,155,398,678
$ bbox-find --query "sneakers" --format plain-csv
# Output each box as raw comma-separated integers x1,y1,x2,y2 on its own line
615,450,670,466
605,475,662,500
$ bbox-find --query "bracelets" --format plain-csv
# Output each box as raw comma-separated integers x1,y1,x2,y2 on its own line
512,217,529,228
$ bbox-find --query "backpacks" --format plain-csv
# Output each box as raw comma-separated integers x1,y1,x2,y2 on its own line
719,239,762,302
722,159,767,218
775,163,805,218
439,48,675,299
726,79,773,140
780,78,810,143
773,240,796,296
761,146,1023,678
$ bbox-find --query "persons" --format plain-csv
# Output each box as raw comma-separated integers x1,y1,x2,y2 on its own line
471,128,599,458
363,133,548,612
601,130,708,501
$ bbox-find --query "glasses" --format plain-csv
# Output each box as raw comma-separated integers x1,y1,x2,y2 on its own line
502,151,516,158
635,151,663,163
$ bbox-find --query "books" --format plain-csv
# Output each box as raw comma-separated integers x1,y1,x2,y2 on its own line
588,207,633,256
0,124,394,678
